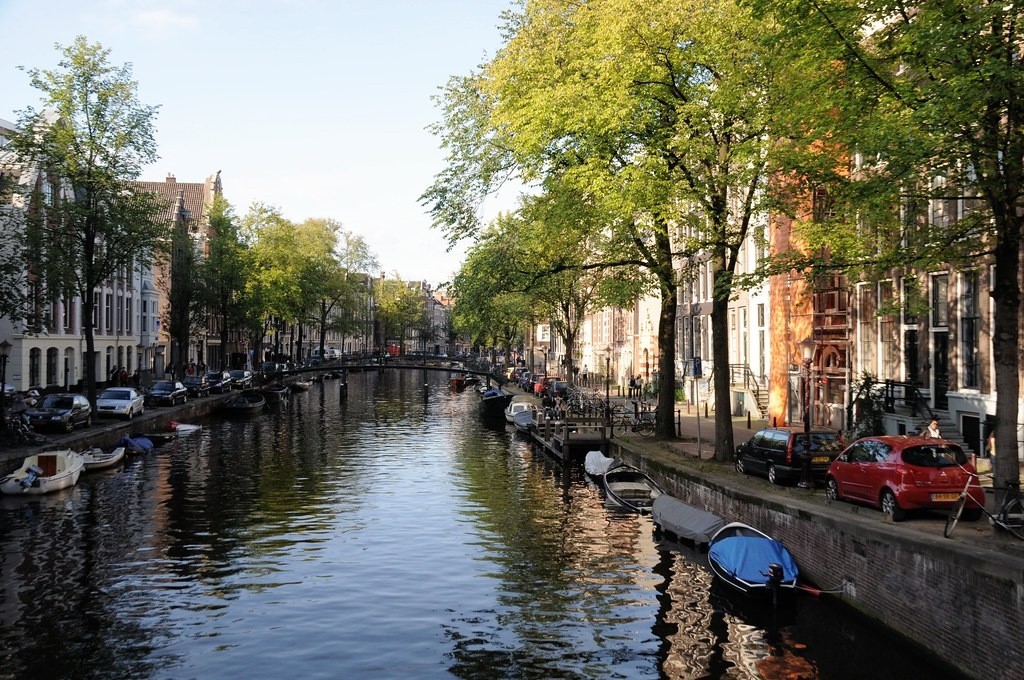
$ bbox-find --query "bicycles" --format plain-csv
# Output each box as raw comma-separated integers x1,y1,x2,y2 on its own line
565,384,656,437
944,458,1024,543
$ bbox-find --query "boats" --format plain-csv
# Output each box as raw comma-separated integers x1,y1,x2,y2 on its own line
120,435,154,454
707,522,799,597
464,371,479,385
512,410,536,434
169,420,203,438
0,447,86,496
449,372,466,388
290,380,314,392
74,445,126,470
652,492,723,546
478,385,488,394
602,453,666,514
132,430,179,444
503,400,535,422
480,388,513,409
219,390,267,416
583,451,614,476
258,384,290,401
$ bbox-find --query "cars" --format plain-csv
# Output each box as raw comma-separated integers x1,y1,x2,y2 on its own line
824,435,985,522
203,371,233,395
546,380,574,400
24,392,93,434
96,387,145,420
0,382,19,404
733,426,847,487
256,345,343,383
228,370,254,390
487,364,561,399
181,376,211,397
146,381,189,407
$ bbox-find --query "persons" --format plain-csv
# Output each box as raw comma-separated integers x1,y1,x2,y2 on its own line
582,365,588,381
988,427,997,476
919,417,942,439
573,363,580,385
185,358,207,376
120,367,128,387
542,393,568,420
133,370,141,388
110,365,119,387
629,374,644,399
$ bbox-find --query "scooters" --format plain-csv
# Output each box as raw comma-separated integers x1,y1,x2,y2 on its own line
3,408,35,448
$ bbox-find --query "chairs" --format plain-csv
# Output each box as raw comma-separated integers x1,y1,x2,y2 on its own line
921,448,937,465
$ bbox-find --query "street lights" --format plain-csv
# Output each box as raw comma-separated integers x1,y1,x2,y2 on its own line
0,338,13,407
512,345,517,384
135,341,146,390
602,343,613,426
541,345,550,388
